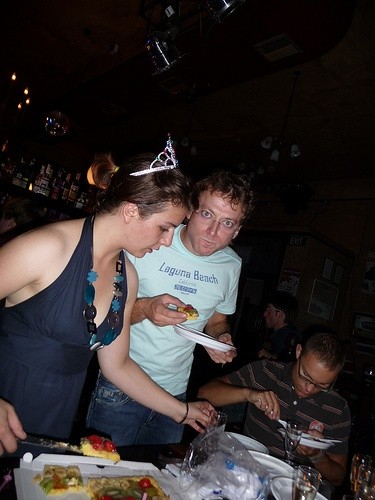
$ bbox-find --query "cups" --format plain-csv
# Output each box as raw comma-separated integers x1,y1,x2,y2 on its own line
349,455,375,500
205,410,227,445
292,465,321,500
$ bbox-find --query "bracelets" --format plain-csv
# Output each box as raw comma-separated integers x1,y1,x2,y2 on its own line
178,403,189,424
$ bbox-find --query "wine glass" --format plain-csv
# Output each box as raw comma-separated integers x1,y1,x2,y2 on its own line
284,423,302,464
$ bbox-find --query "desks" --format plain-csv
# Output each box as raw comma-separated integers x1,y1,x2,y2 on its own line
0,443,189,500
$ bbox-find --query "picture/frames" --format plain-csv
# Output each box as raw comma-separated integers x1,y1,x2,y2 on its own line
320,257,346,286
307,278,340,320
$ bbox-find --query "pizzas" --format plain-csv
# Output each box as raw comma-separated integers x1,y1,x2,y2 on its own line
178,304,199,319
89,475,171,500
33,465,84,495
80,435,120,462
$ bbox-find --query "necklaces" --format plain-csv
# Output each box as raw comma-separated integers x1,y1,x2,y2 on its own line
84,253,124,349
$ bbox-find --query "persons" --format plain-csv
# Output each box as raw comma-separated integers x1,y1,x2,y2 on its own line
197,331,352,486
258,291,306,363
0,131,220,457
86,172,254,449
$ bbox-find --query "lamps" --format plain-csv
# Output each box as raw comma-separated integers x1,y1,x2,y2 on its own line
206,0,246,23
146,17,180,74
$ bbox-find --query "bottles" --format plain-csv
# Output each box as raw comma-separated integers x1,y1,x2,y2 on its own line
0,139,85,209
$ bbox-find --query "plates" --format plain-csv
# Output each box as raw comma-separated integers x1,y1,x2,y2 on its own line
226,432,269,454
173,324,236,353
277,427,334,449
247,450,294,481
270,476,327,500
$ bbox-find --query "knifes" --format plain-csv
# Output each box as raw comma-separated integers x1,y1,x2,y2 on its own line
15,434,82,454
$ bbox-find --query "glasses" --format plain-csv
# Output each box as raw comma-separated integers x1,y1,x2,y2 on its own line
265,307,281,313
298,353,338,393
